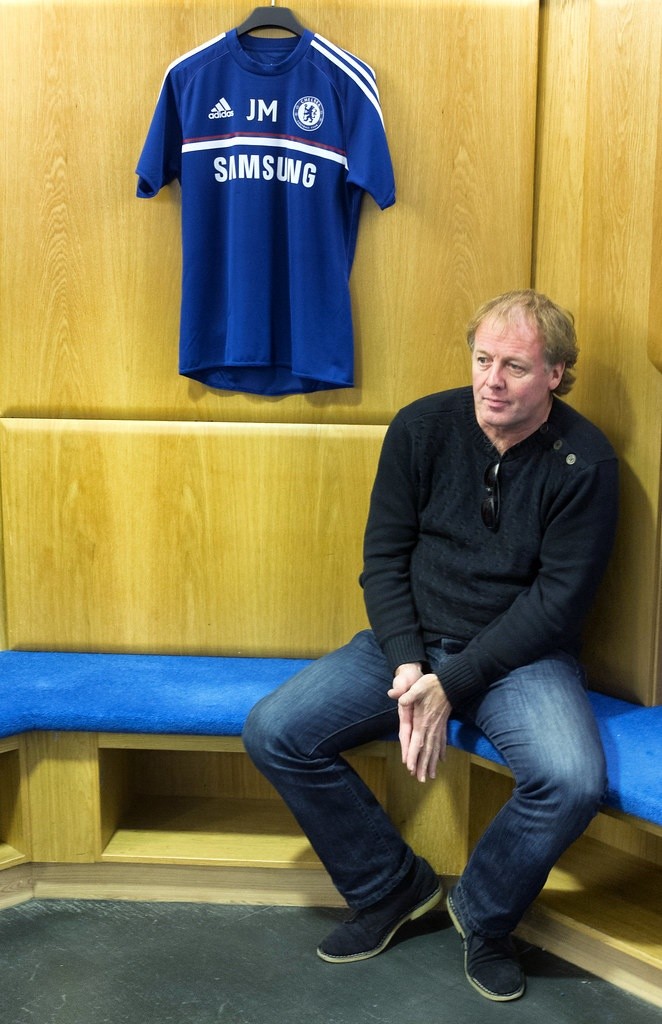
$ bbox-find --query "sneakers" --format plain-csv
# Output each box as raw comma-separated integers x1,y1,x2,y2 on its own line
315,854,445,964
446,890,525,1001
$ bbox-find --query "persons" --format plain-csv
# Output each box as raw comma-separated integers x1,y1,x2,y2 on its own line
243,291,619,1001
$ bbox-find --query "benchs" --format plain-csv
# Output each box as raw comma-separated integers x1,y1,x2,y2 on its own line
0,650,662,1013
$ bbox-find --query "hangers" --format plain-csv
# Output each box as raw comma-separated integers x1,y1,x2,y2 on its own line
161,0,377,86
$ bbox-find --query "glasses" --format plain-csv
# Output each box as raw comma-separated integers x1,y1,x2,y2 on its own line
480,459,502,533
